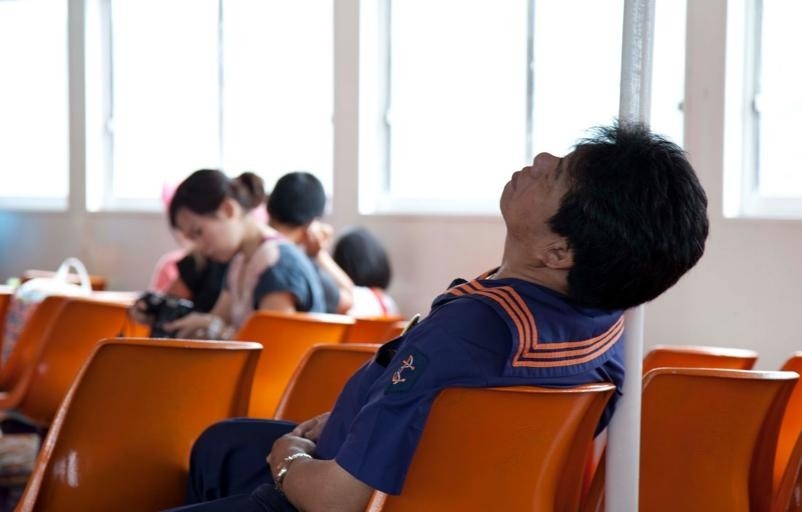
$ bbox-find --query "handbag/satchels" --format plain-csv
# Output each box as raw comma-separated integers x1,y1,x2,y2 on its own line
2,258,93,370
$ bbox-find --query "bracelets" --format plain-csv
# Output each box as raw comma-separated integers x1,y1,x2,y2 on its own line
206,316,224,340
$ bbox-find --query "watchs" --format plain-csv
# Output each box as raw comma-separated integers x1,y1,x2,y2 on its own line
272,452,312,496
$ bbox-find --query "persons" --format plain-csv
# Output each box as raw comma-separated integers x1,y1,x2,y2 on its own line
129,168,328,341
148,180,228,315
182,119,710,512
331,229,401,319
265,171,354,317
